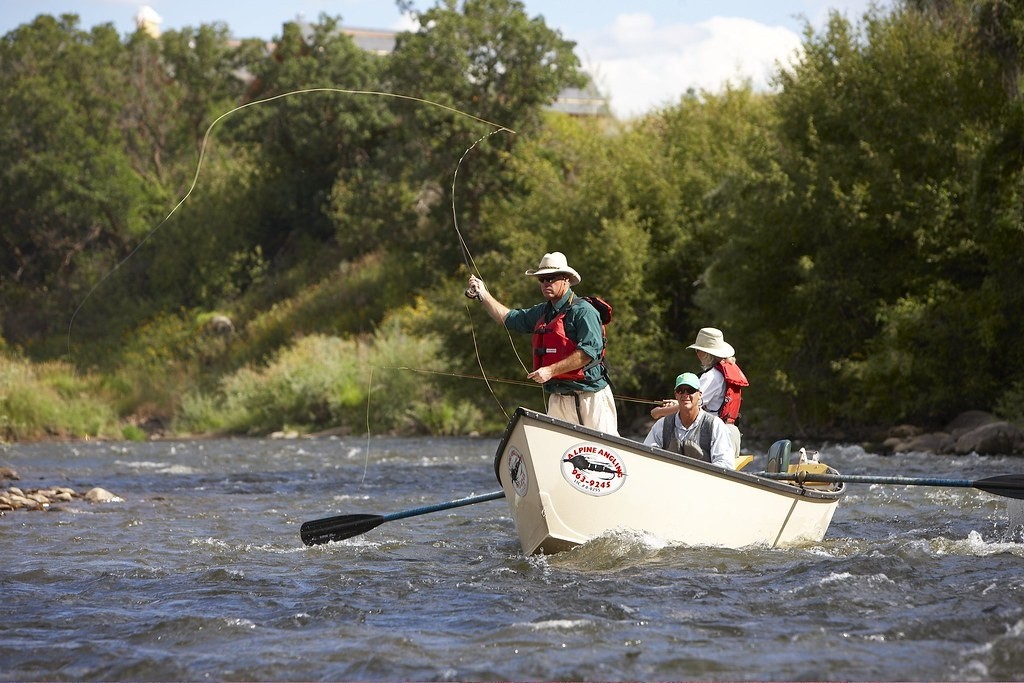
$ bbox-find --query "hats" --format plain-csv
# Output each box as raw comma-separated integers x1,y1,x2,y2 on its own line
686,328,735,358
525,252,581,286
674,372,701,391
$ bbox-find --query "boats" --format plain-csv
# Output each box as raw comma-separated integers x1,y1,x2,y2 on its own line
491,405,847,559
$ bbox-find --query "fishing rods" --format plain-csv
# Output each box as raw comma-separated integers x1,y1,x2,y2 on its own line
450,126,506,302
378,365,667,407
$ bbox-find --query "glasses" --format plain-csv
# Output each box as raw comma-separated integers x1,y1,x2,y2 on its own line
696,349,702,353
676,388,698,394
539,274,563,283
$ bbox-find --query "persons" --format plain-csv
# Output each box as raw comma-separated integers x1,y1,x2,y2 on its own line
643,372,737,471
468,251,622,438
651,327,750,427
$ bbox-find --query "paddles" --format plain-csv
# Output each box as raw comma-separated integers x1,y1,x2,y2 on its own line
753,471,1024,501
298,490,506,548
